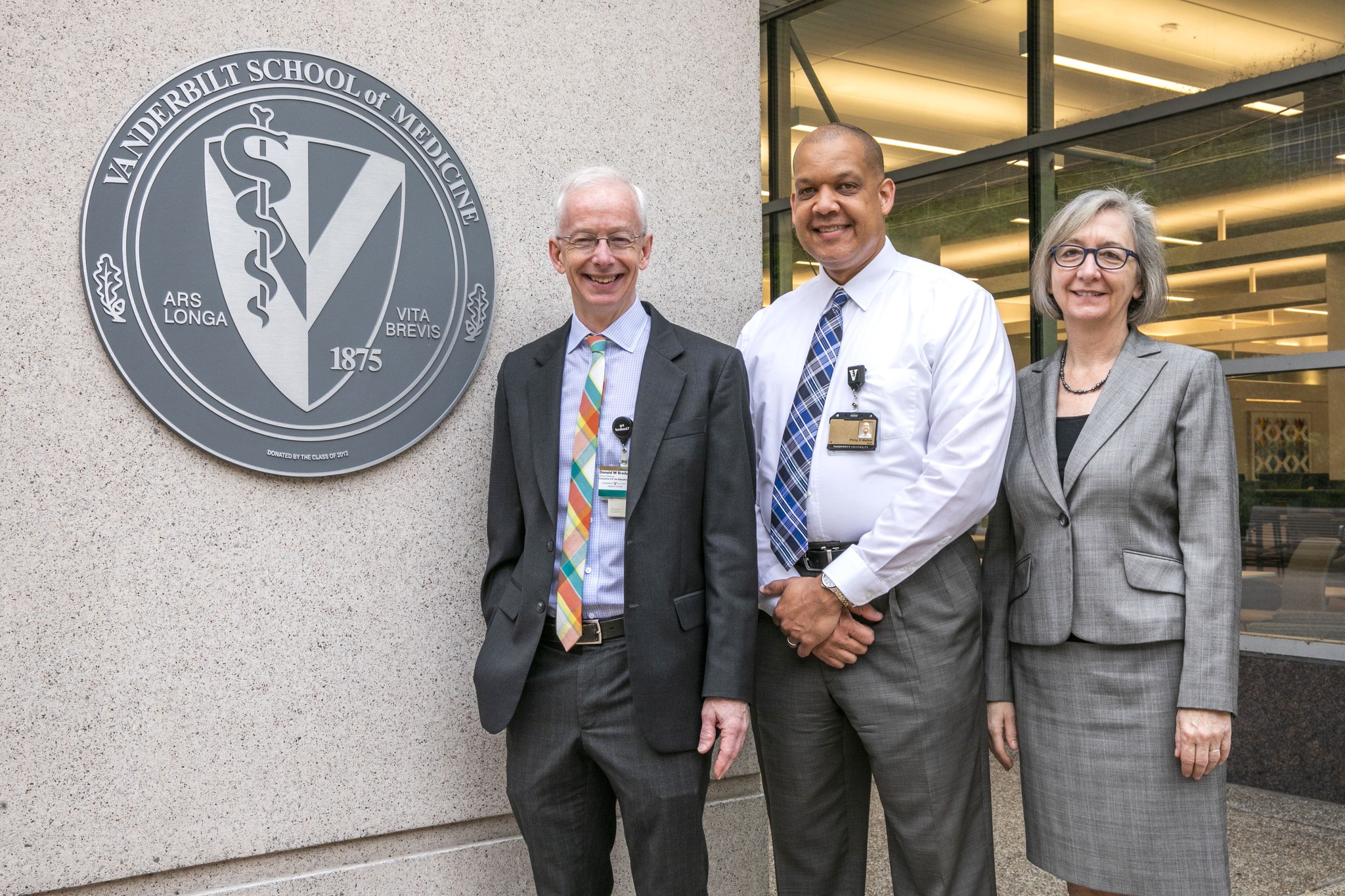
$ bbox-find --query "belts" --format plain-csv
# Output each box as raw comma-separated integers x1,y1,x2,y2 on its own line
542,615,624,646
791,542,854,572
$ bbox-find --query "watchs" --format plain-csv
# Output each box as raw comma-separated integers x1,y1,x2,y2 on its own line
820,571,851,607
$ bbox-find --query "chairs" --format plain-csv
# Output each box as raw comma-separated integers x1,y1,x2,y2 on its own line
1238,536,1342,633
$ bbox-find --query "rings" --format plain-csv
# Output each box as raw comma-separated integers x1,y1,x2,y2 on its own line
786,636,797,646
1209,749,1220,752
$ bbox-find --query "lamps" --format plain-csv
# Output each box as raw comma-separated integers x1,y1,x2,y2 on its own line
1019,30,1305,117
789,71,1064,171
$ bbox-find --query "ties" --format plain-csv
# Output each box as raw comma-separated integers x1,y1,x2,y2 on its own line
553,333,610,653
769,287,856,578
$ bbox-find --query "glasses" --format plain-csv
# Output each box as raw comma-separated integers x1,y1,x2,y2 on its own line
1049,244,1141,270
557,232,647,249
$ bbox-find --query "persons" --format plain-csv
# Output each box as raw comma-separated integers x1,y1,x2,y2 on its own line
982,188,1241,896
471,165,759,896
735,123,1018,896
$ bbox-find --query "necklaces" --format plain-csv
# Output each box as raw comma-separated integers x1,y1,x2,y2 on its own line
1060,345,1111,395
